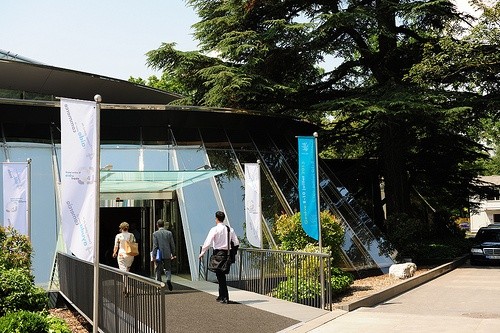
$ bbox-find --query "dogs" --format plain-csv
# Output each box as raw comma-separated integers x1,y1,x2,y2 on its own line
389,262,417,279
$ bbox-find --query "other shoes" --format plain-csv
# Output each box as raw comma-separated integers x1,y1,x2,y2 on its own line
216,297,229,304
167,280,173,291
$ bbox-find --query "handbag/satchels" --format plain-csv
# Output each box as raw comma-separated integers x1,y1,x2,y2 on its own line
125,233,139,256
208,252,229,274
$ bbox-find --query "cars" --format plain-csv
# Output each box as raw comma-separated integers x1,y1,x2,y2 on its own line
470,226,500,265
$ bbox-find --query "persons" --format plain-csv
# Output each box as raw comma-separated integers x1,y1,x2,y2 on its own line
198,210,239,304
129,223,141,274
152,218,177,291
112,221,137,298
